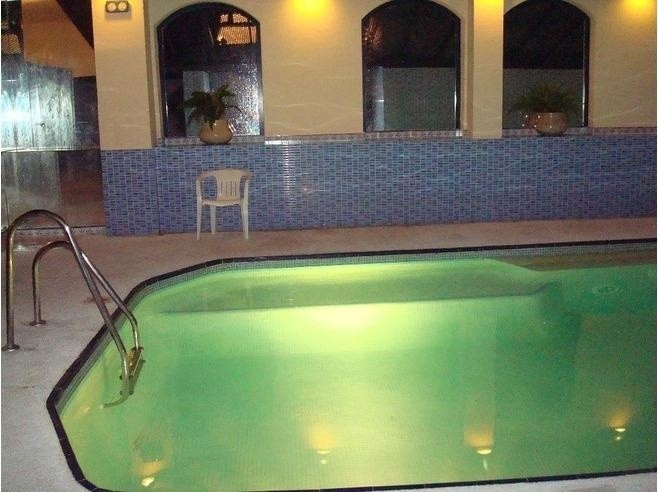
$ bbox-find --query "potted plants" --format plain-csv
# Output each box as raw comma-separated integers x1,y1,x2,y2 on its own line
507,78,583,137
176,85,244,145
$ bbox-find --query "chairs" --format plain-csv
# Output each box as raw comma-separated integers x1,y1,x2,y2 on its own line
195,168,253,240
